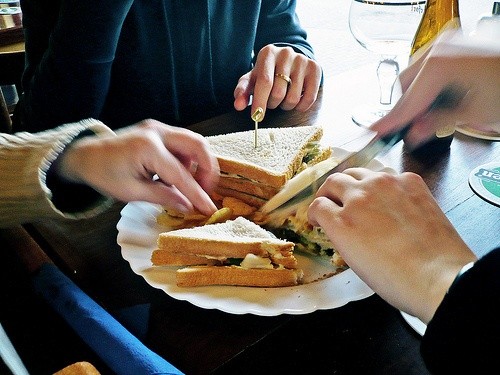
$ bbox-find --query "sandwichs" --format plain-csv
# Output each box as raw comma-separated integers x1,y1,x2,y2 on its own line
250,158,352,265
201,126,333,207
150,217,301,286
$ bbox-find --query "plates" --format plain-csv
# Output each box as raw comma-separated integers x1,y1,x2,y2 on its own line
115,147,398,316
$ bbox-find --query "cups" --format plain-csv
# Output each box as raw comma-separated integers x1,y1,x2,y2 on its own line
0,83,19,117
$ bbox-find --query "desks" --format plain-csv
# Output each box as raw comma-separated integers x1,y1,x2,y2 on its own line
22,56,500,375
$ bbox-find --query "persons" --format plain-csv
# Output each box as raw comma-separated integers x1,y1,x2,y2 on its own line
0,118,220,220
11,0,324,133
307,20,500,375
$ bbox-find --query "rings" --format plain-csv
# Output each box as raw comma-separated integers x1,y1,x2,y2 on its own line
275,73,290,83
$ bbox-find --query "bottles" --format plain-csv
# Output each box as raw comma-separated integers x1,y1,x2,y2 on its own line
409,0,461,150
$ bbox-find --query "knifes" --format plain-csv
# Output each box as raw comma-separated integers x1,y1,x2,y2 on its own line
267,124,410,216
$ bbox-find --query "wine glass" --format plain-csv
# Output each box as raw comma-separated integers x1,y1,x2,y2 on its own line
349,1,428,129
